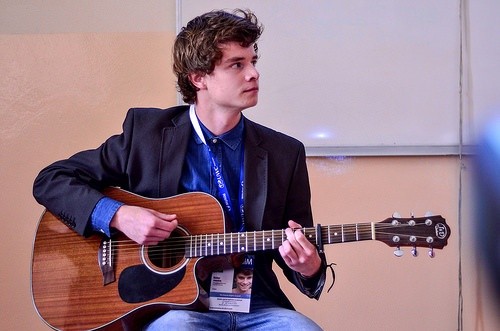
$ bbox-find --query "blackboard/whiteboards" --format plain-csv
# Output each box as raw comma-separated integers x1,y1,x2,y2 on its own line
176,1,500,157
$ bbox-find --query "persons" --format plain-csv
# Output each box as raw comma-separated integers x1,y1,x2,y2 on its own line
231,265,252,296
32,7,326,330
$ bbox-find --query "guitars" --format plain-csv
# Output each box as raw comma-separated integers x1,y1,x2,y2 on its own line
31,185,452,331
